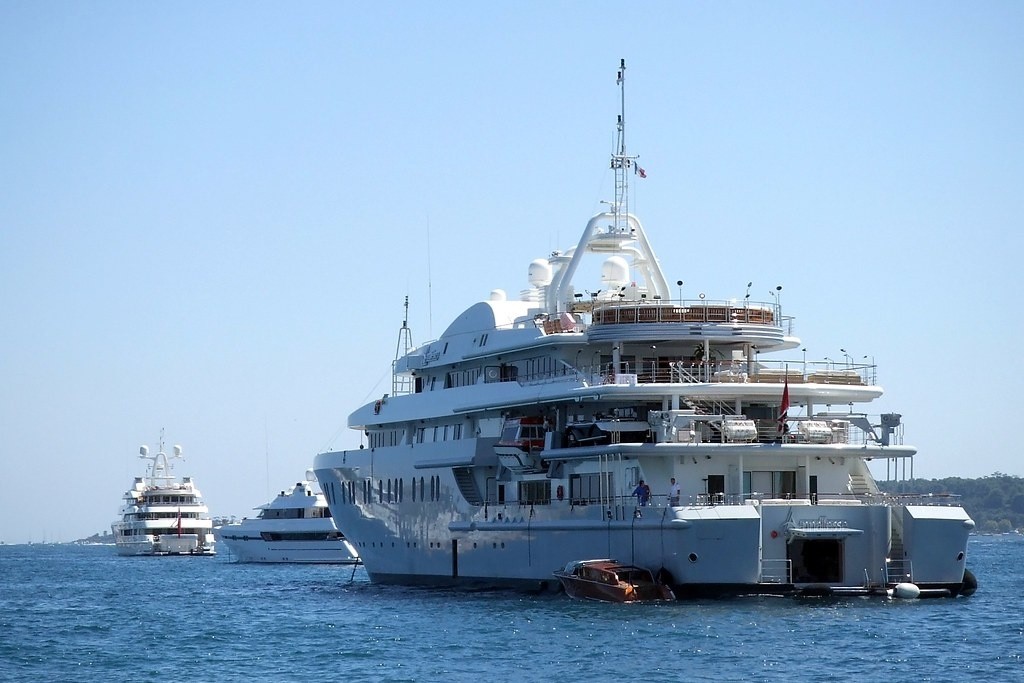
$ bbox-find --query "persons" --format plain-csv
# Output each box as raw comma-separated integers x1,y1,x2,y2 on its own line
667,478,681,507
631,480,650,506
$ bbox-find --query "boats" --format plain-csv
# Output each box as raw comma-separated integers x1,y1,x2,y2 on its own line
111,428,217,556
553,559,675,603
219,469,364,564
315,59,978,598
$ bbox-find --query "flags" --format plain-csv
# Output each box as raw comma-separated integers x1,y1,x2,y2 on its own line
635,162,646,178
777,375,790,432
